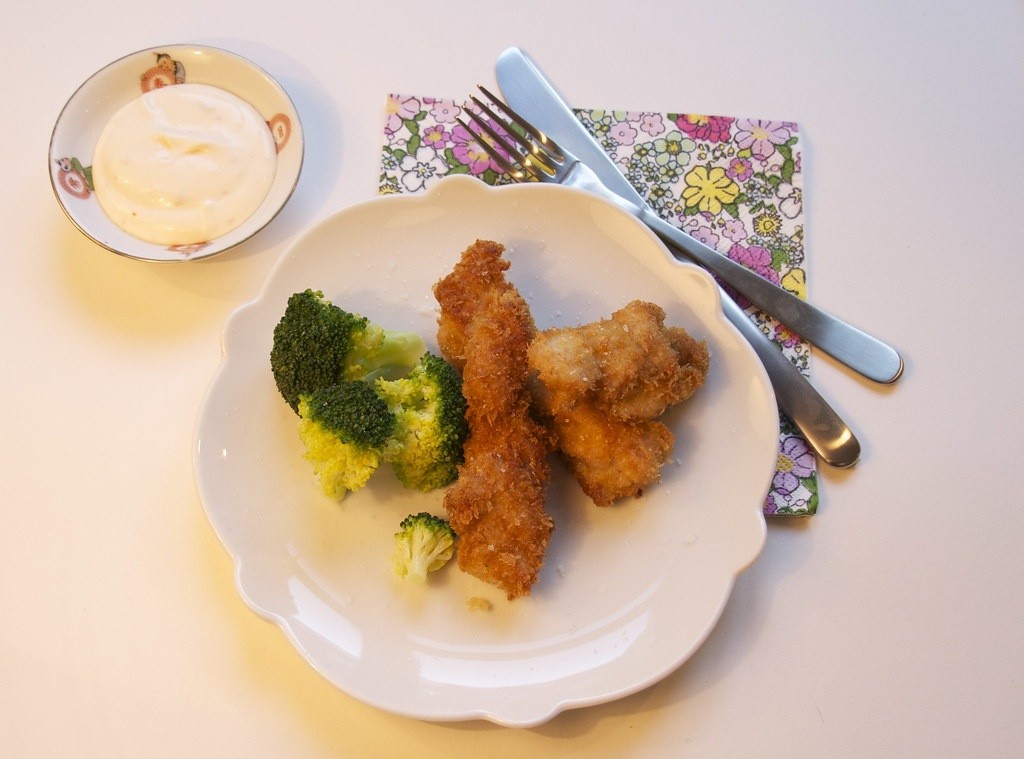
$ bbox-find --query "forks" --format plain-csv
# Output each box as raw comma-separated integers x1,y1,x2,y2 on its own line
454,85,904,386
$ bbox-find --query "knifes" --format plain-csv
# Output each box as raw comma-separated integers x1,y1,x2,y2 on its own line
493,46,862,470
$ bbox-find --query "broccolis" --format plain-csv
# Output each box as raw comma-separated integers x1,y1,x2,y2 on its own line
269,290,465,583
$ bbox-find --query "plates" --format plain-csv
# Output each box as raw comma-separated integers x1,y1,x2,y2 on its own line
189,170,782,729
47,43,305,264
